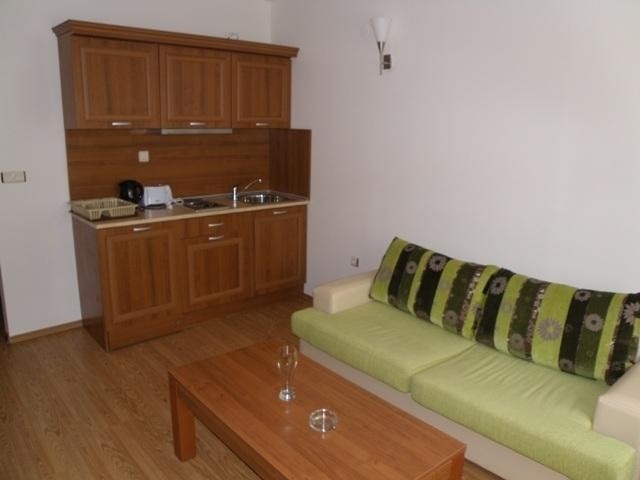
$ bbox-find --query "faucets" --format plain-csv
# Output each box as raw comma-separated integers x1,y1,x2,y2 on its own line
231,176,263,201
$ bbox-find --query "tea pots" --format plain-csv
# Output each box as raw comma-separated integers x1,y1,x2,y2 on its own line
118,180,143,203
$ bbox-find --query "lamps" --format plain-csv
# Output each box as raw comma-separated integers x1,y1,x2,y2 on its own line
370,16,392,75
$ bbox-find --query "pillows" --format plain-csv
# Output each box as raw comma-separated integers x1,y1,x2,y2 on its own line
473,273,640,386
369,237,498,338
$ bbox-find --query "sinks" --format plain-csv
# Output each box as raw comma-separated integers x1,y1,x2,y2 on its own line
236,190,285,206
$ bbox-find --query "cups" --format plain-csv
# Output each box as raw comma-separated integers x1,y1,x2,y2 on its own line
277,345,299,401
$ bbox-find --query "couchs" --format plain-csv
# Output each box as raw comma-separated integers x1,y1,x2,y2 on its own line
290,273,640,480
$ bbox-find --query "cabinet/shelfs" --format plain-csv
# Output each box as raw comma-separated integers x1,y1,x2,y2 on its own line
98,205,306,352
76,35,160,125
160,44,233,130
233,50,292,130
